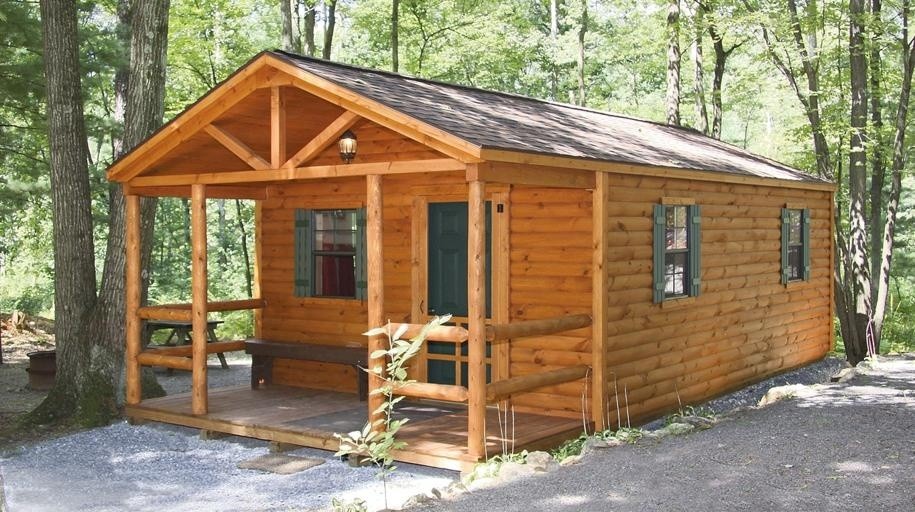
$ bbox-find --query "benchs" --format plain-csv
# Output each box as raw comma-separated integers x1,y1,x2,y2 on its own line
244,337,368,401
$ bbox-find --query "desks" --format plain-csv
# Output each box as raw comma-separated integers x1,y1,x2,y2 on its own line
145,321,228,369
